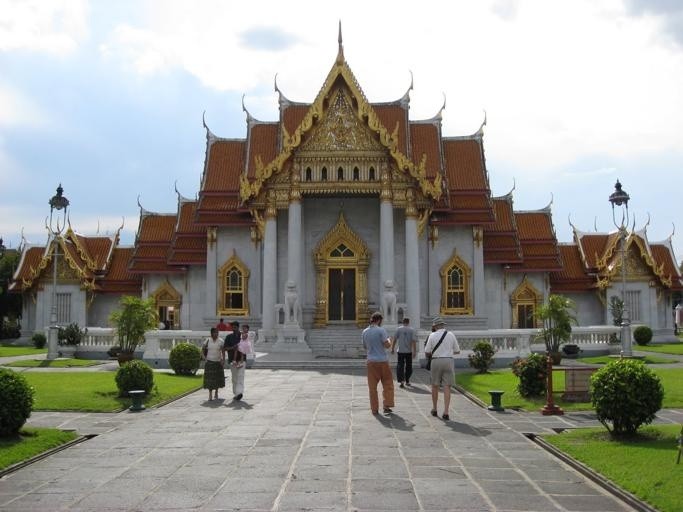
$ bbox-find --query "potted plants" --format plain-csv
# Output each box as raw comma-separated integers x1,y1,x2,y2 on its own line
54,322,88,359
531,294,580,365
107,294,160,368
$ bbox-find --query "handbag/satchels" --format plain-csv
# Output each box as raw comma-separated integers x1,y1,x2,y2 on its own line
419,358,432,371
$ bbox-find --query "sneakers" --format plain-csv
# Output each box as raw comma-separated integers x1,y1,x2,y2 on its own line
372,406,394,416
430,409,438,417
399,381,413,388
234,393,244,402
443,413,450,421
230,361,244,368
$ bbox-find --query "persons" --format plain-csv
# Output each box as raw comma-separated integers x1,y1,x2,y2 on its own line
424,326,442,387
390,319,417,387
216,318,226,331
224,322,246,401
202,328,225,401
226,322,233,330
229,325,251,368
361,312,394,414
424,317,460,420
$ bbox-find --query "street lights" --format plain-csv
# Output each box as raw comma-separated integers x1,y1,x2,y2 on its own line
608,178,646,358
34,183,70,361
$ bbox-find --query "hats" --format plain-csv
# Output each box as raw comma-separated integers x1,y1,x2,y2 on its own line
432,316,448,329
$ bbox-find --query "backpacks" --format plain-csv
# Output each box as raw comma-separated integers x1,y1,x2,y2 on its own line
202,339,210,360
